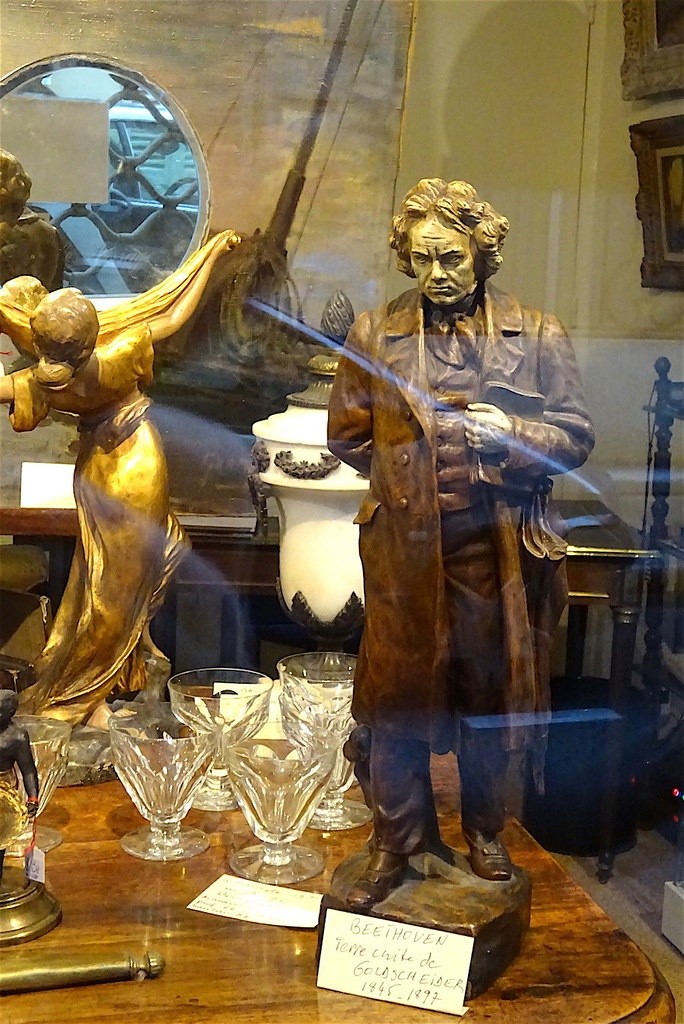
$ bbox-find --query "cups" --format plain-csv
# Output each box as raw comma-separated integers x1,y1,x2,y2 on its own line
166,667,274,812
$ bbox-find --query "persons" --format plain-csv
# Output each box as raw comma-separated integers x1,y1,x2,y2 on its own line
0,229,240,740
327,179,596,909
0,148,66,291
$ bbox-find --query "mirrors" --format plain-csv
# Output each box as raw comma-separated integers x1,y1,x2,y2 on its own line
0,51,212,318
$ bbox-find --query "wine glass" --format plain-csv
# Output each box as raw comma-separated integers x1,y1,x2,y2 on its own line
221,722,342,885
13,715,73,854
107,702,218,862
277,651,375,831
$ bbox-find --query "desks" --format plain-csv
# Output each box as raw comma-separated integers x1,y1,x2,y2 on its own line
153,500,662,883
0,692,677,1024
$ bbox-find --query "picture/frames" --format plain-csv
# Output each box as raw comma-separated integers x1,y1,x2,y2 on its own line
628,113,684,291
620,0,684,101
